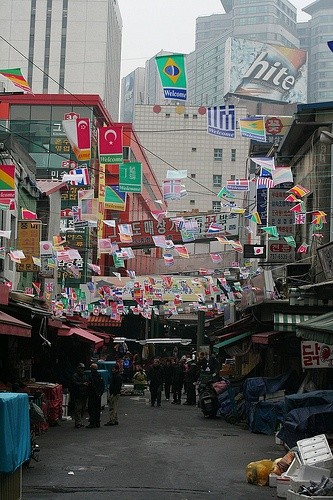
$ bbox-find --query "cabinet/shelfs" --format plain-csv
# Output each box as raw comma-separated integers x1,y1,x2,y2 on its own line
86,392,108,410
0,382,62,500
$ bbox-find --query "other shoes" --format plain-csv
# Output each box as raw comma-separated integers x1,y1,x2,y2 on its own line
85,424,95,428
79,424,84,427
95,422,100,427
104,421,112,426
75,424,80,428
112,421,118,425
150,391,195,408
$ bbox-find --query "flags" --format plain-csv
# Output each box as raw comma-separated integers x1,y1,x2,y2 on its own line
1,53,327,318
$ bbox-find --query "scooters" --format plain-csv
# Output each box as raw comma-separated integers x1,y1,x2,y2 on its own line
192,375,222,419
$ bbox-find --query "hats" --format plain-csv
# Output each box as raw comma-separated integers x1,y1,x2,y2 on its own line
89,363,98,369
77,362,85,367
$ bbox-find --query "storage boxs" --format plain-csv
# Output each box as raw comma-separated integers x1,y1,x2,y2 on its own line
246,390,285,401
269,434,333,500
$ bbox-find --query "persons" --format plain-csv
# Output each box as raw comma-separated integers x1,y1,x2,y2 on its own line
90,345,227,408
70,363,89,428
104,365,123,426
86,363,105,428
